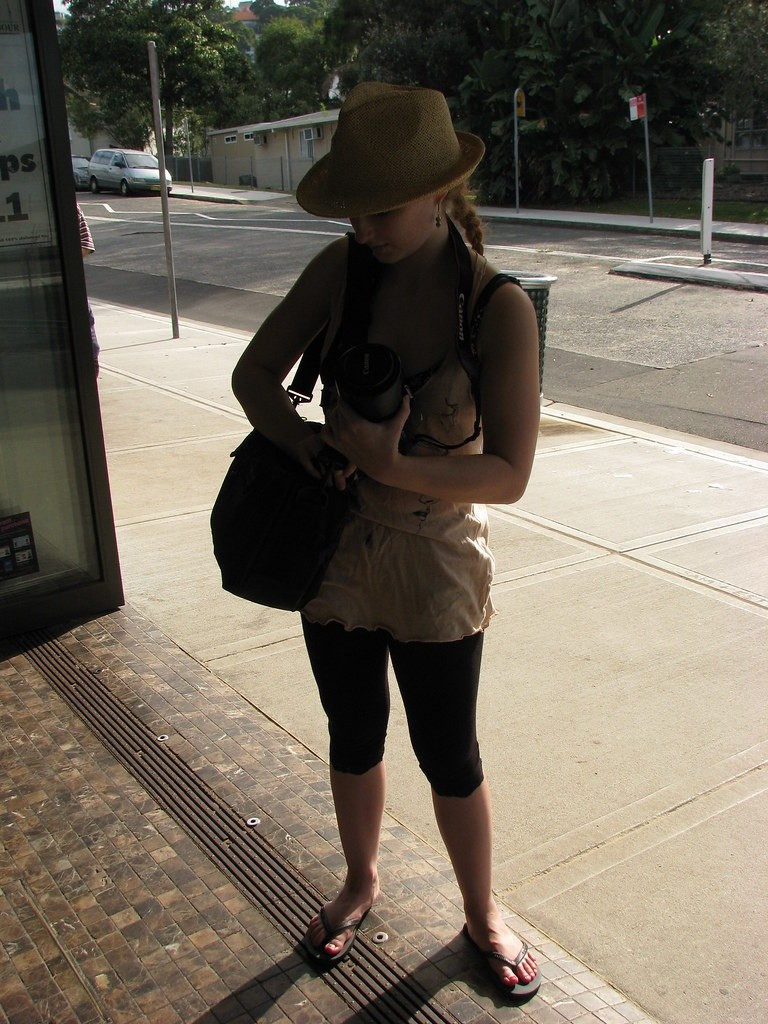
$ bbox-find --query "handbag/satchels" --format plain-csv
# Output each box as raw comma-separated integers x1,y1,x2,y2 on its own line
211,421,361,610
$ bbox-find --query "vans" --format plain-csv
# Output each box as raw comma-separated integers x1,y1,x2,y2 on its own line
89,149,173,197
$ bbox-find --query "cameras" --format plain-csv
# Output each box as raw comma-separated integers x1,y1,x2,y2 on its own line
331,344,417,486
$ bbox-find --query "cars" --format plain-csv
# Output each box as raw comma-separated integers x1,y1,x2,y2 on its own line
71,155,90,192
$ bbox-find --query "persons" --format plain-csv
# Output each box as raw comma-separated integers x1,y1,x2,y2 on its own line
231,83,543,997
76,201,100,379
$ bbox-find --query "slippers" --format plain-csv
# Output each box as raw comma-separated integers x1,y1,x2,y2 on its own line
302,892,373,963
463,924,542,997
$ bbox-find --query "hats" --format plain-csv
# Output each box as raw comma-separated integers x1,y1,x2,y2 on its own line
297,82,486,218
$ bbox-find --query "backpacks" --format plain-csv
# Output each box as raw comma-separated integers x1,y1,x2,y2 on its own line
468,273,549,392
